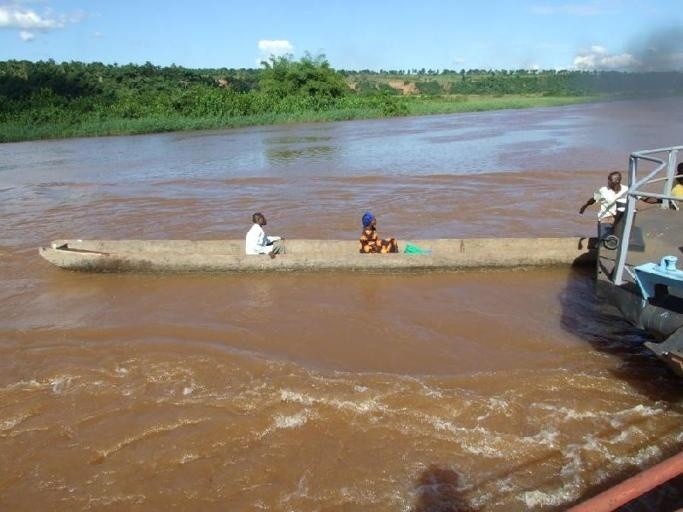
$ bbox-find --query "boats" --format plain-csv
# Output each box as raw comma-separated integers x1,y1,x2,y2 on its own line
39,238,598,275
596,145,683,379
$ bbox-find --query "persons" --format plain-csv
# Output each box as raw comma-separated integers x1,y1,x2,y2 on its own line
245,212,286,258
669,162,683,200
579,171,662,242
358,212,397,255
